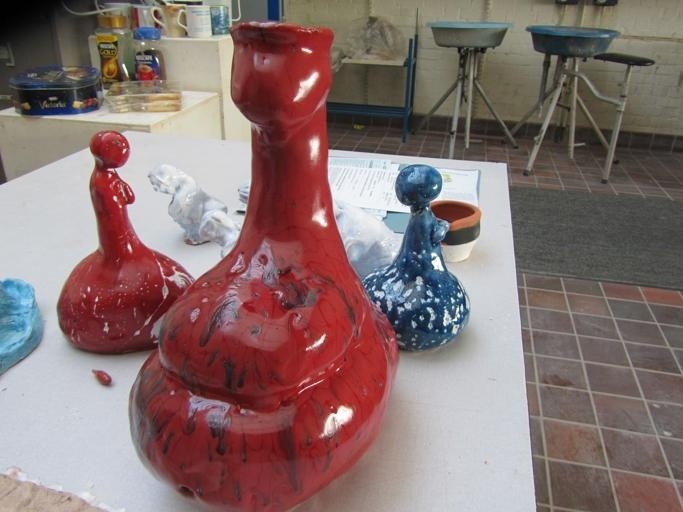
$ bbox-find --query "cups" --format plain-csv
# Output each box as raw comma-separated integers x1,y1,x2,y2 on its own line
151,0,241,38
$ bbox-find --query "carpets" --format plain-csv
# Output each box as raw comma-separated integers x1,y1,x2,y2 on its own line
509,182,681,292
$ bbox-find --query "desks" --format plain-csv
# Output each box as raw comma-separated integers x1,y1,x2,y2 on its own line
0,132,535,512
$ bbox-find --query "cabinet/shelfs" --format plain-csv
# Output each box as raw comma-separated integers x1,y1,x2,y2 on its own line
322,40,417,144
